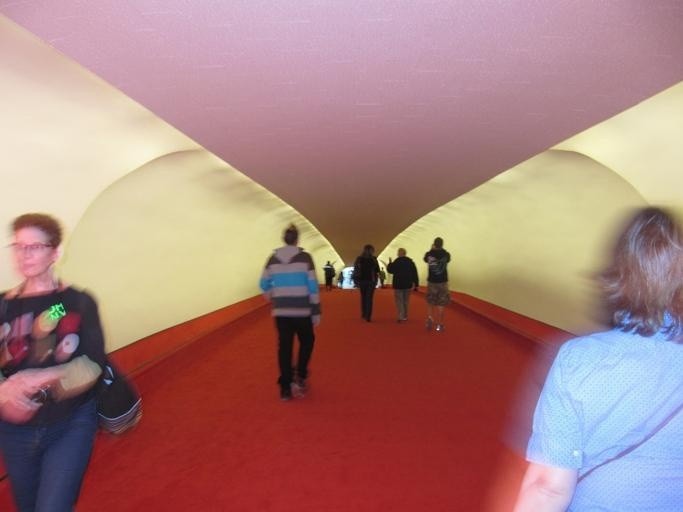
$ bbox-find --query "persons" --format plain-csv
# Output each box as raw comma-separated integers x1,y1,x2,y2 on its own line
259,223,321,403
0,211,106,512
379,267,386,287
350,244,380,323
323,260,332,291
511,205,682,512
423,237,450,334
337,270,344,288
384,246,419,321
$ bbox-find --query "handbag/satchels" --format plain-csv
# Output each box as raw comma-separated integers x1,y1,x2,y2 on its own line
95,365,141,434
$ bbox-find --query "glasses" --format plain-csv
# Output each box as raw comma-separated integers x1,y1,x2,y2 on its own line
12,243,54,250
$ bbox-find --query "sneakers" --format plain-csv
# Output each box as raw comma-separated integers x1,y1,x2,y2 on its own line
426,316,434,329
292,375,305,387
281,388,290,399
436,324,443,331
